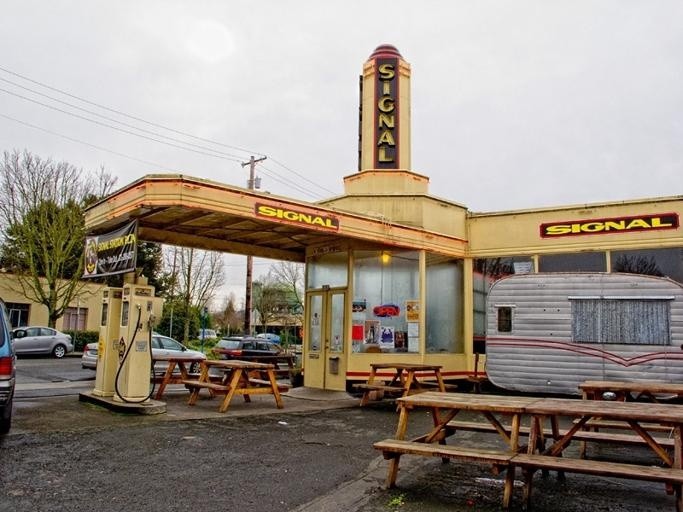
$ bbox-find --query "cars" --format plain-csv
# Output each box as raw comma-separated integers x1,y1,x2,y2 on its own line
256,331,280,343
10,325,74,357
198,329,218,339
83,333,208,380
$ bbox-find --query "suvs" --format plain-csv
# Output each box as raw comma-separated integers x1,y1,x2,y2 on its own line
1,297,14,433
213,335,298,364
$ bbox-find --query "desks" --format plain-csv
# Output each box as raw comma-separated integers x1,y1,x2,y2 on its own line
384,390,683,512
188,359,284,412
152,356,216,400
578,380,683,459
360,362,446,413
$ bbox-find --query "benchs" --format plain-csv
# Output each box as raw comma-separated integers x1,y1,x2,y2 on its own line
180,379,231,396
572,418,675,434
372,438,683,485
248,378,290,393
445,420,674,449
418,381,458,388
352,384,407,392
171,372,223,382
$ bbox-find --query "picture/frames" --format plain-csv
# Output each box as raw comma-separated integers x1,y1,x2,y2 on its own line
365,319,381,345
405,299,420,323
